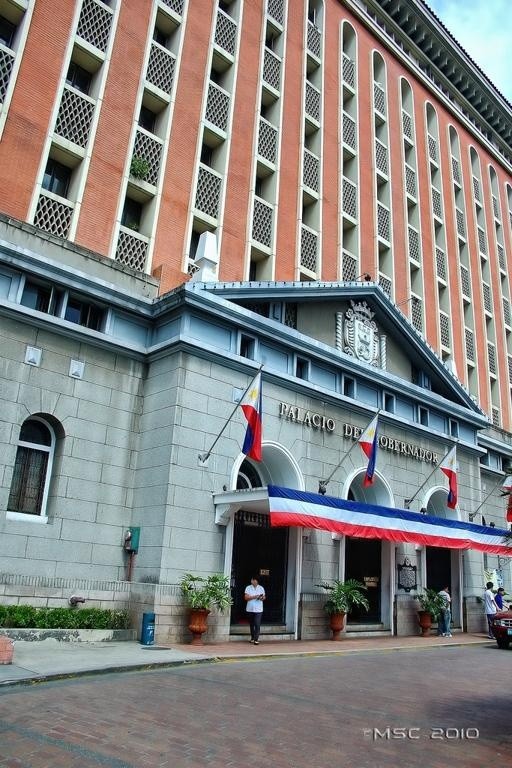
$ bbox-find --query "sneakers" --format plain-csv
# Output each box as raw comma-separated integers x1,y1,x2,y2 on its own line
249,639,255,643
438,633,453,638
254,640,259,645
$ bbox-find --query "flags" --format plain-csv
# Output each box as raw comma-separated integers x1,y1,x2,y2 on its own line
359,414,380,487
505,466,512,526
242,366,262,465
439,444,458,509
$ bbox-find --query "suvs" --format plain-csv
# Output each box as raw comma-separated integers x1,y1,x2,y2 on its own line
491,604,512,650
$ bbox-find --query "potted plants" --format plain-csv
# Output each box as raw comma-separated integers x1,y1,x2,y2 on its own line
312,577,370,641
413,587,450,637
175,572,234,646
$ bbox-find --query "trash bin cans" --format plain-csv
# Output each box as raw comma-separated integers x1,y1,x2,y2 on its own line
141,613,155,645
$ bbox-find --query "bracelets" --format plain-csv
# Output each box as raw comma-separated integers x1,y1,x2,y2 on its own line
260,597,264,600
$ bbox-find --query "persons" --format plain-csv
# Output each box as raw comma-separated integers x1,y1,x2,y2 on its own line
485,582,502,638
243,574,268,645
493,588,510,612
435,591,445,637
433,584,453,637
